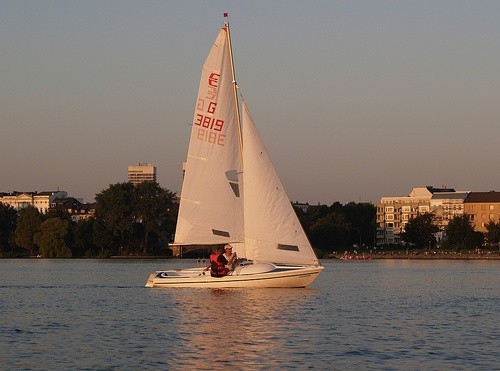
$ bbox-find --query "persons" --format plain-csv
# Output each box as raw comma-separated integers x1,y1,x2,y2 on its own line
204,245,238,271
342,248,498,259
210,246,236,278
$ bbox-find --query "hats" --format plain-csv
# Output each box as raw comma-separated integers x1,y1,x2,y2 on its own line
224,245,234,249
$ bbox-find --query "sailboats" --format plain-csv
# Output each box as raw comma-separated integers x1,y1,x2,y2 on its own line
145,12,325,289
